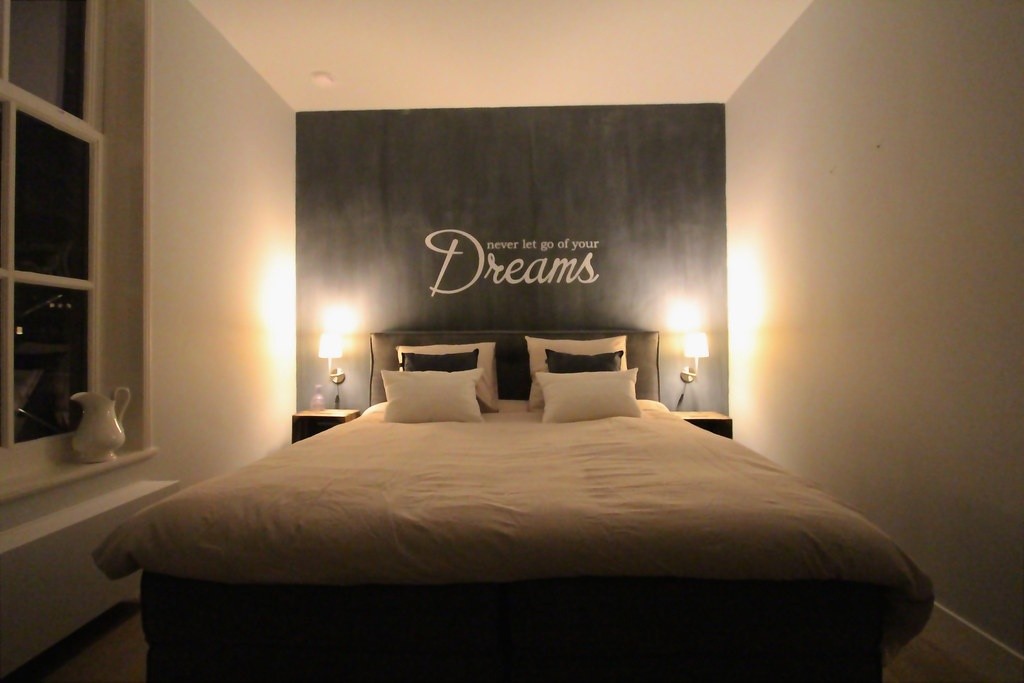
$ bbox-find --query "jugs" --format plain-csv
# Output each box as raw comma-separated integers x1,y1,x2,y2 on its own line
68,386,132,463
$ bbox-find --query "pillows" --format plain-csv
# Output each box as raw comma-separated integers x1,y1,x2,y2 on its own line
524,335,628,412
380,368,485,423
402,348,479,373
396,342,499,414
536,367,643,424
545,348,624,373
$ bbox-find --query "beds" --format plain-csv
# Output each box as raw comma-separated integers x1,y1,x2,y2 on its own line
91,329,934,681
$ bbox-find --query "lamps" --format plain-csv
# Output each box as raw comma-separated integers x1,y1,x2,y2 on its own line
679,331,709,383
316,333,346,384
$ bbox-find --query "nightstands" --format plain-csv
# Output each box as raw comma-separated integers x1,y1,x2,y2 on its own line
669,410,733,439
292,408,361,444
